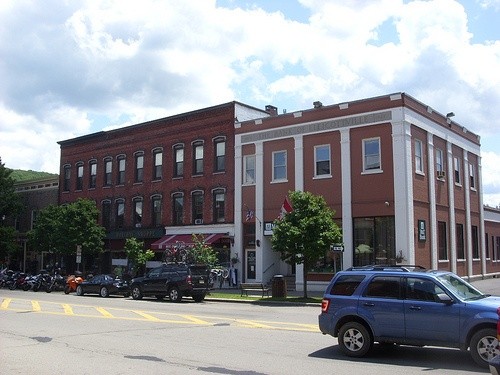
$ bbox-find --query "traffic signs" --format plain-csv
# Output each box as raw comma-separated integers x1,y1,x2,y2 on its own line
333,245,344,252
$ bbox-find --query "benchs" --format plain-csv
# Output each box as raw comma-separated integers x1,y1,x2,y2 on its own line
240,283,270,298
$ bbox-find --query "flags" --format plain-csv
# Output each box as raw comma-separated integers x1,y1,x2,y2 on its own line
277,196,294,221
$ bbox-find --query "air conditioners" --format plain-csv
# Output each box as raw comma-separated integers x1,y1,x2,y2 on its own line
437,171,446,177
195,219,203,224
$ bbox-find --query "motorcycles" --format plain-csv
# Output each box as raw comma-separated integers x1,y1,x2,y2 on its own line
0,267,94,294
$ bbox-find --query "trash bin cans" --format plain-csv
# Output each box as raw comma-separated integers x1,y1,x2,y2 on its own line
271,274,287,300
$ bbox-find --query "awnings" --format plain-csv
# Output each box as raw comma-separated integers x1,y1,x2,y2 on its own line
150,234,225,250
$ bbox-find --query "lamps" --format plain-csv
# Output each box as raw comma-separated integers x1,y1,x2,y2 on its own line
446,112,455,118
313,101,322,109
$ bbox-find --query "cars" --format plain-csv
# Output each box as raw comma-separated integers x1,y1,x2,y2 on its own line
76,273,130,298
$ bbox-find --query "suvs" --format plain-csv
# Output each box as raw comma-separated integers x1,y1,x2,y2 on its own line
128,262,211,303
318,264,500,370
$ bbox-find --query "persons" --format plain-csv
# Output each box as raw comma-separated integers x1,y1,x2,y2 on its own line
416,281,441,300
